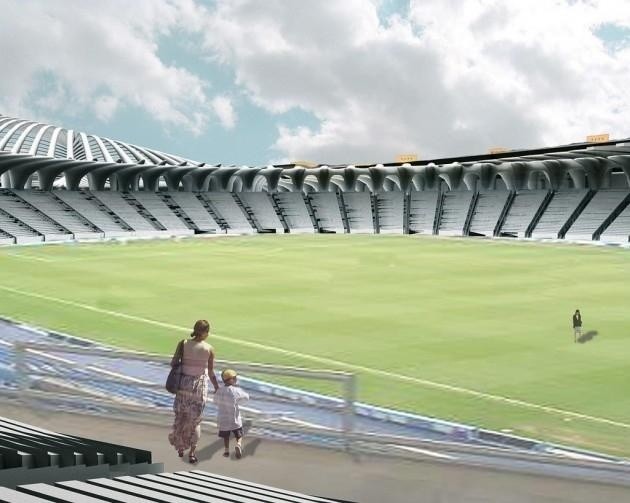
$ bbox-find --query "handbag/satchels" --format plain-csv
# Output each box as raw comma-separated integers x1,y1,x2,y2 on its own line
165,338,185,394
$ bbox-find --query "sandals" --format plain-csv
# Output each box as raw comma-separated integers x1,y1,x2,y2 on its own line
178,450,184,457
189,455,200,464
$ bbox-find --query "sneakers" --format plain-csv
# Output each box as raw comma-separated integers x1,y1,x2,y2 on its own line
234,445,242,458
222,452,229,457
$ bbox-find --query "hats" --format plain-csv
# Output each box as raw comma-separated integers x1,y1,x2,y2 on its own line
221,368,241,382
